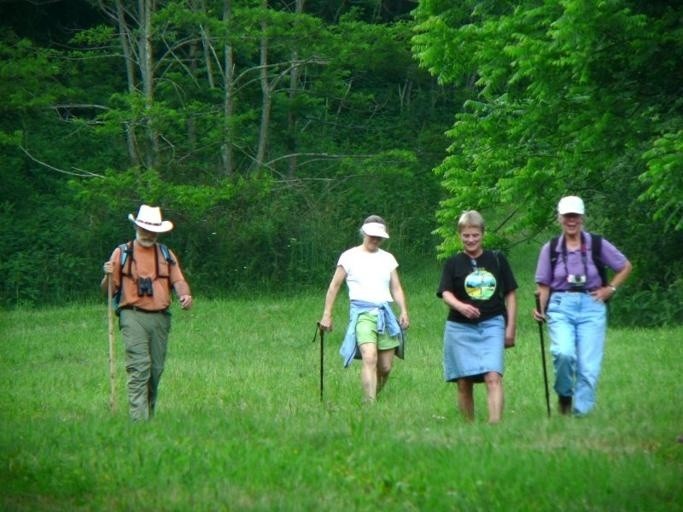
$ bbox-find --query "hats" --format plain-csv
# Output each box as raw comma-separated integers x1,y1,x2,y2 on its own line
362,222,390,239
559,196,584,214
128,204,174,233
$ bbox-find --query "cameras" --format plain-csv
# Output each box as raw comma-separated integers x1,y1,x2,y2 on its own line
566,274,586,287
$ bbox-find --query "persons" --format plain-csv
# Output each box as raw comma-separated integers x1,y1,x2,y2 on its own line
533,195,633,418
319,214,411,399
100,204,193,422
435,209,519,424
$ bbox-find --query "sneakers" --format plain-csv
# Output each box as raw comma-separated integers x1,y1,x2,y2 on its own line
559,396,571,414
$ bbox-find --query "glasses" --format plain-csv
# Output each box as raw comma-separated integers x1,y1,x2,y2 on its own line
471,259,478,272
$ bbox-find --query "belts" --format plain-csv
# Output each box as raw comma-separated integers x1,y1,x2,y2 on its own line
123,306,168,313
554,289,592,293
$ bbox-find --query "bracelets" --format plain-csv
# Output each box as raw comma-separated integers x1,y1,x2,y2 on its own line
606,283,616,292
